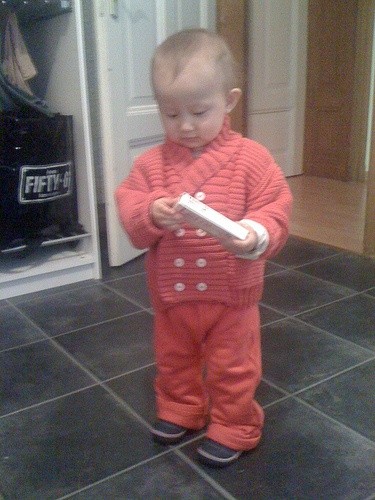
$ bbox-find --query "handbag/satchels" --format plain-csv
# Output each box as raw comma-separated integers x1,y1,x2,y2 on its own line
0,109,85,259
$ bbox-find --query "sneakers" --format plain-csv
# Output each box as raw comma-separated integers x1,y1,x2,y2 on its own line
150,420,187,443
195,436,242,467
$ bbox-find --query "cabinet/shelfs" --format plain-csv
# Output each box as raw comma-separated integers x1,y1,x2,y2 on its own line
0,0,102,302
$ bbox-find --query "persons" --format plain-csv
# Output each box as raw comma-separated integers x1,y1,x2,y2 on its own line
112,27,296,467
